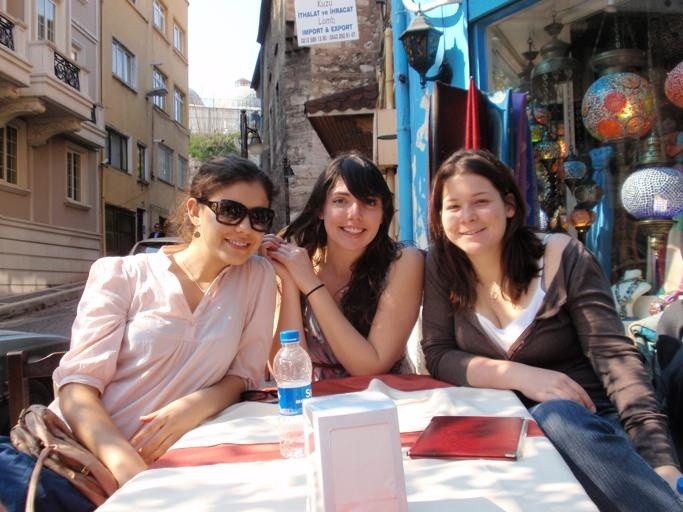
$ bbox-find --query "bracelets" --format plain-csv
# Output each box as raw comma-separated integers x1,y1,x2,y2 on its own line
306,283,325,298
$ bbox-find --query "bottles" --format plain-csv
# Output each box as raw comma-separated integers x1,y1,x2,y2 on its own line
273,330,314,458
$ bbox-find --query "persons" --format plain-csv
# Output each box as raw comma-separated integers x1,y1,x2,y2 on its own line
149,223,166,238
258,150,424,381
1,156,279,512
423,146,682,512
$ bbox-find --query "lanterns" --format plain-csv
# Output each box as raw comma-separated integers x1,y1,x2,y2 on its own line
524,62,683,260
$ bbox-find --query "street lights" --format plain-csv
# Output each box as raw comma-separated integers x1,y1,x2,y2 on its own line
240,109,264,158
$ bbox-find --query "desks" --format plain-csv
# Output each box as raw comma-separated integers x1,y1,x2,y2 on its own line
89,374,603,511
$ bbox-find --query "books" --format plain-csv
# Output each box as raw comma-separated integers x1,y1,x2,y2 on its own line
407,416,528,461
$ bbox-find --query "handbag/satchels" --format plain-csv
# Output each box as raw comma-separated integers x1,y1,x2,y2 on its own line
11,404,119,507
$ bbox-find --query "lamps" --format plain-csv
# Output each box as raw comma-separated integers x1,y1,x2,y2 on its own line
398,2,454,88
581,3,663,150
664,56,683,112
515,16,605,238
618,130,683,262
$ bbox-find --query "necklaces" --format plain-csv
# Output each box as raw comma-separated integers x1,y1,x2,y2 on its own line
179,256,206,294
614,277,638,321
476,277,503,299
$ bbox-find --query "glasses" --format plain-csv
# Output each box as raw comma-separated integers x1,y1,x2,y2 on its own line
240,390,278,400
197,198,274,231
